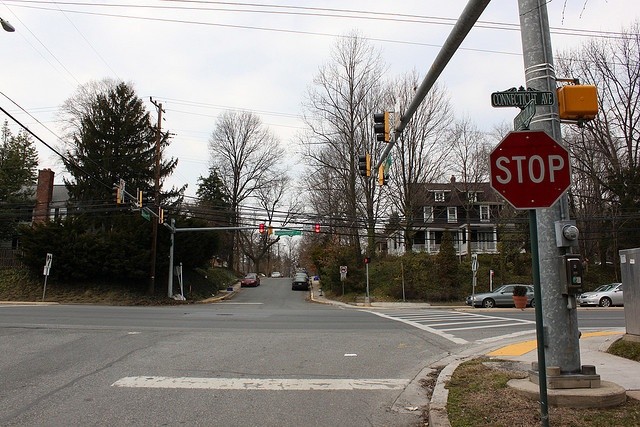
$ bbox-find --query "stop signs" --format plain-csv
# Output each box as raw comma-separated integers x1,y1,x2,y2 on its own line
487,130,572,210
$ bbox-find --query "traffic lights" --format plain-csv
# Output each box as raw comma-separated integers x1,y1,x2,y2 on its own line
158,207,172,226
112,186,121,205
374,111,394,145
364,257,371,263
314,222,321,233
139,190,148,210
357,154,374,179
259,223,264,235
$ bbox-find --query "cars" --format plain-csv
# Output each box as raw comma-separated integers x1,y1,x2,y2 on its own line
292,275,309,292
579,282,624,307
258,272,266,277
241,272,260,288
292,272,309,281
576,285,608,308
466,285,536,308
271,271,282,278
